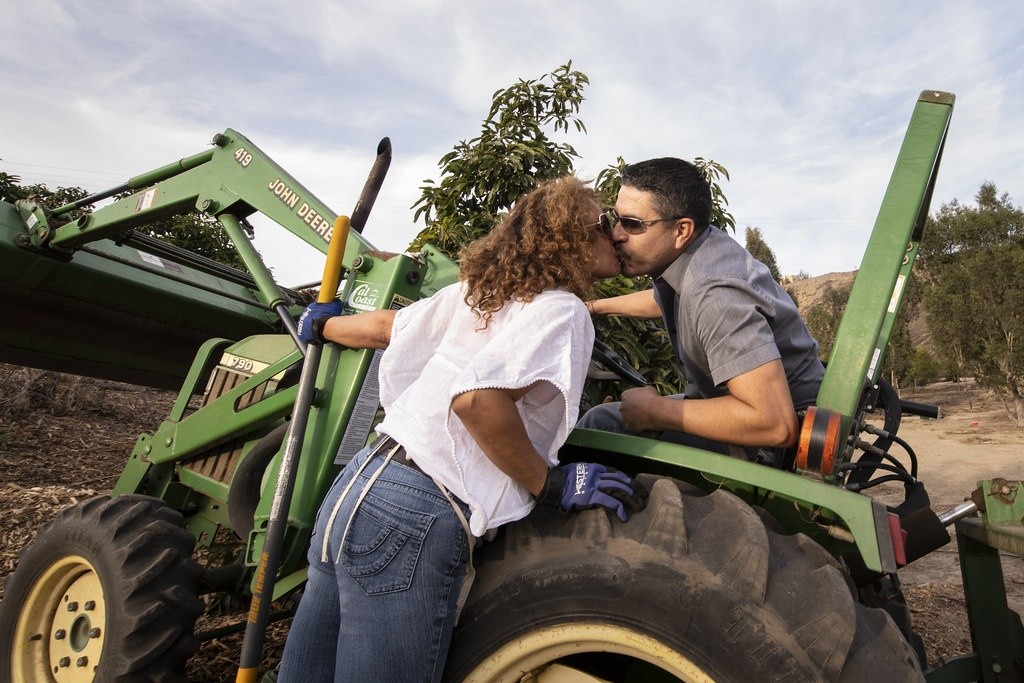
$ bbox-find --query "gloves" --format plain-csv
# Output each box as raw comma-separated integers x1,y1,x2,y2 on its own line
531,462,649,522
297,298,343,344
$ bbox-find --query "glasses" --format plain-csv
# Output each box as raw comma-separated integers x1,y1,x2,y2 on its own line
584,213,611,236
611,210,698,235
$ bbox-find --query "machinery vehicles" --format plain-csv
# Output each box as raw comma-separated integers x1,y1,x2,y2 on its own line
0,87,1024,683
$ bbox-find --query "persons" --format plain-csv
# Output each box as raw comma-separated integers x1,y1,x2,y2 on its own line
277,173,645,683
575,157,827,470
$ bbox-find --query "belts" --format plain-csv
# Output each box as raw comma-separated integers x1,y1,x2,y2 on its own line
371,433,449,493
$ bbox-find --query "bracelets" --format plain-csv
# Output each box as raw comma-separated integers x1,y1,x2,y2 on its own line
591,301,595,314
